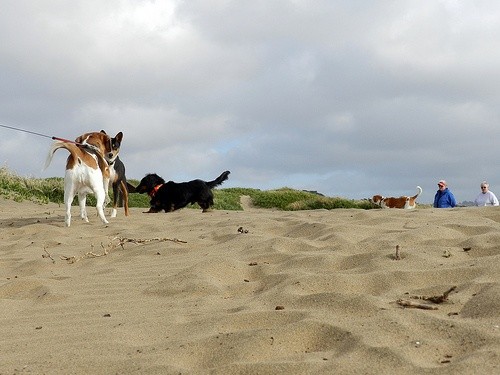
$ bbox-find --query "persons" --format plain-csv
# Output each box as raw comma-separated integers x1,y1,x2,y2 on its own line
475,181,499,206
433,180,456,209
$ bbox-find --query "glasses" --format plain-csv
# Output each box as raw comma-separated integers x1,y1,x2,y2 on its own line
439,186,443,187
481,187,486,188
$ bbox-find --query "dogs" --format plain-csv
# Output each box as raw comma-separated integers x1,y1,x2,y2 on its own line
44,131,112,227
100,130,137,218
136,170,230,213
372,186,422,210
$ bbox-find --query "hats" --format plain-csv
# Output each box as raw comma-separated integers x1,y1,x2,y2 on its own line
437,181,447,187
